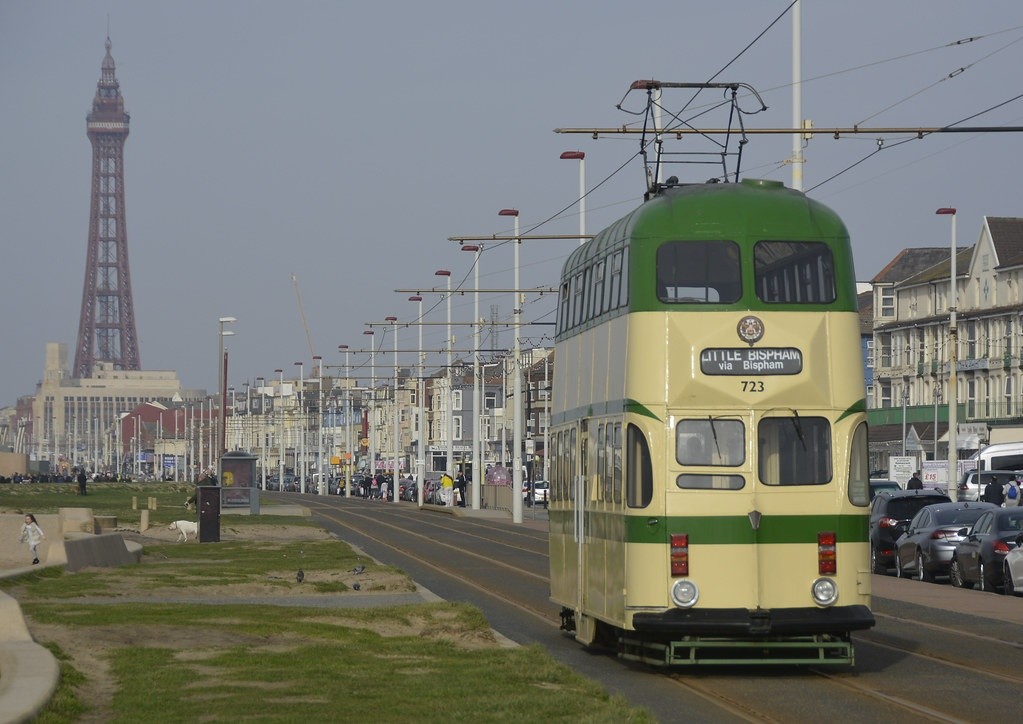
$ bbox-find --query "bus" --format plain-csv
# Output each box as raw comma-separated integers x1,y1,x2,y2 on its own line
547,178,876,667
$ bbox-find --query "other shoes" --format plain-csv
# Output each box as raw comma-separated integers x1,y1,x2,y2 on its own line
460,505,465,507
33,558,39,564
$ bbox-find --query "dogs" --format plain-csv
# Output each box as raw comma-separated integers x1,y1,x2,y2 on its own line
167,520,198,543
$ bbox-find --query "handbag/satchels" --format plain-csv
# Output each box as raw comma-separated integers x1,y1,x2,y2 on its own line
372,480,377,486
337,488,340,494
388,492,392,496
453,488,459,493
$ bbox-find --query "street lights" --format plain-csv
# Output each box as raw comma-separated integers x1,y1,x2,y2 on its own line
363,331,375,477
313,356,323,495
228,377,266,490
461,245,480,510
498,209,524,523
275,369,284,492
294,362,305,493
36,396,212,482
217,317,237,485
338,345,351,498
385,317,400,502
435,270,453,480
630,79,664,187
408,297,424,506
935,208,958,503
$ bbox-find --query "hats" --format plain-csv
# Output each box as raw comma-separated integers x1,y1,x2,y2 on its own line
458,470,462,472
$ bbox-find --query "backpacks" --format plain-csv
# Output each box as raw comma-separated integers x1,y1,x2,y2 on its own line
1008,482,1017,499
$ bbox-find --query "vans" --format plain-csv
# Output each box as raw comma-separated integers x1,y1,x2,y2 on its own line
967,441,1023,471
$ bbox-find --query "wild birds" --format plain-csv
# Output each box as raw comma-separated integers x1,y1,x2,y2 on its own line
296,568,304,581
353,581,361,591
348,566,366,575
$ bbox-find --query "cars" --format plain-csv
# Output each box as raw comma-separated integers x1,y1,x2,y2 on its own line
870,468,1023,596
522,480,550,505
257,471,462,506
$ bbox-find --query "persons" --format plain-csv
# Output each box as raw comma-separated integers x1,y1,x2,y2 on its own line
14,473,64,483
187,469,216,505
361,472,393,499
457,471,466,508
408,474,413,480
339,477,347,495
78,471,87,495
985,475,1004,507
19,514,45,564
1002,475,1020,507
95,475,132,482
441,473,454,507
907,473,923,490
